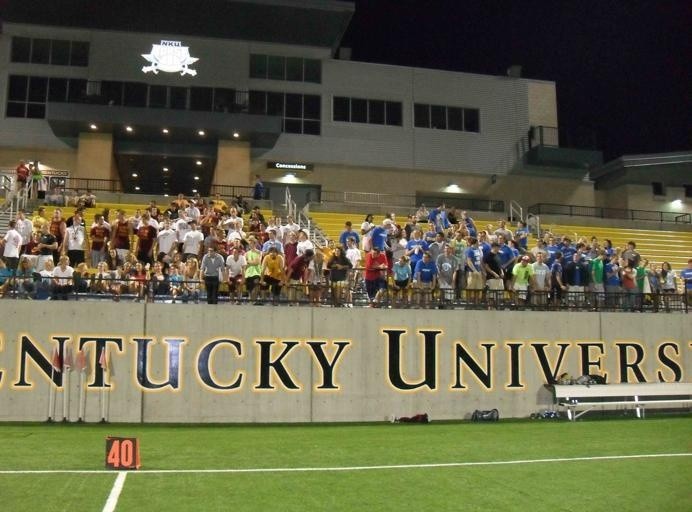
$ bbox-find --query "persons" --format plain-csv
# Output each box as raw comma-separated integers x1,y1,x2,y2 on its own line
2,189,362,308
15,157,30,195
26,163,35,183
361,203,677,312
247,174,264,198
680,260,691,312
31,160,44,200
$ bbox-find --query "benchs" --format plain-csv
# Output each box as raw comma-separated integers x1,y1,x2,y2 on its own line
552,381,691,422
